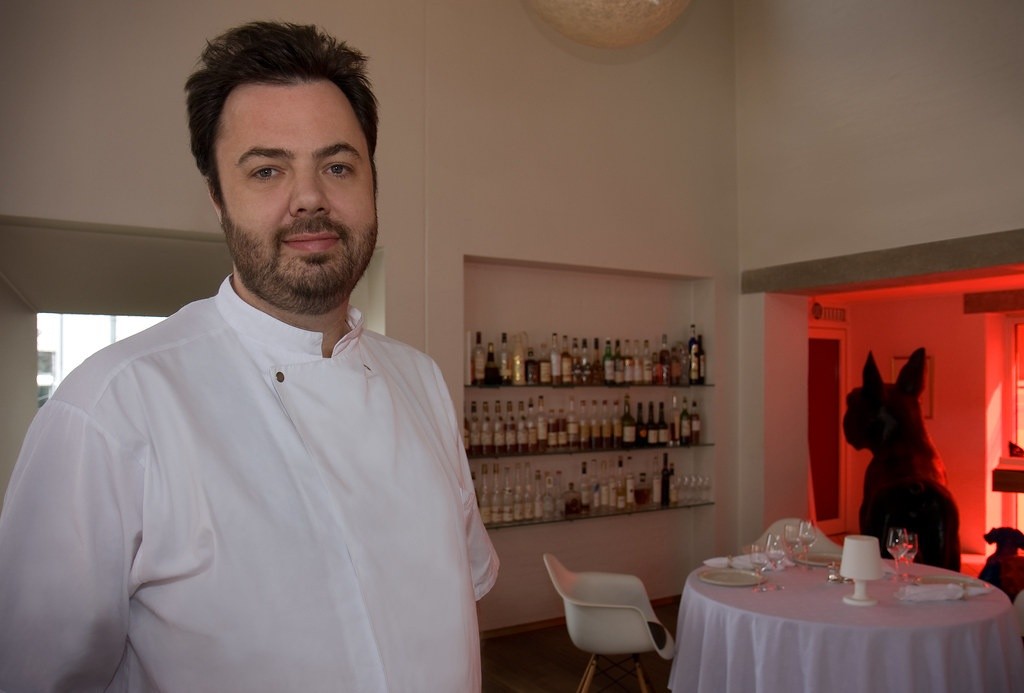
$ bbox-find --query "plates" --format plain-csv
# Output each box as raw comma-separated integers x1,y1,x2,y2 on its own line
694,569,767,589
794,550,843,569
915,574,987,589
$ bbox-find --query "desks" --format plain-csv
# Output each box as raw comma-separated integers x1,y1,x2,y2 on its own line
668,554,1024,693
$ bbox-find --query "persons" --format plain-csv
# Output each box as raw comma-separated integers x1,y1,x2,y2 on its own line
0,22,499,693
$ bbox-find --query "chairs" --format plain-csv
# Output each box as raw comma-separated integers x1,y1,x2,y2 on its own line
743,517,844,556
543,552,676,693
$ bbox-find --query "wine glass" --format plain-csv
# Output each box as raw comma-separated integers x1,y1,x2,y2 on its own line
753,518,818,594
889,527,919,584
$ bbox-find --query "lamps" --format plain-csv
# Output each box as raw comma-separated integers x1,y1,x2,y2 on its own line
840,536,885,606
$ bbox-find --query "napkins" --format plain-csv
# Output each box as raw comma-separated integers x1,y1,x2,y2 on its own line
891,584,997,602
706,551,797,571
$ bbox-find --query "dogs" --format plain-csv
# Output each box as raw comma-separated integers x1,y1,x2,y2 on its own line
843,347,961,573
977,528,1024,604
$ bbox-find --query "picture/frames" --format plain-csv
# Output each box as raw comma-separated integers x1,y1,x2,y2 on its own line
890,353,934,418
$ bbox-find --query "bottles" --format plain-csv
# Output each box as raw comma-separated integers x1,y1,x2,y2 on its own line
465,321,713,527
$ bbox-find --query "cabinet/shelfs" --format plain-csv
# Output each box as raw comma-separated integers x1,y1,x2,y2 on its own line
464,382,717,530
